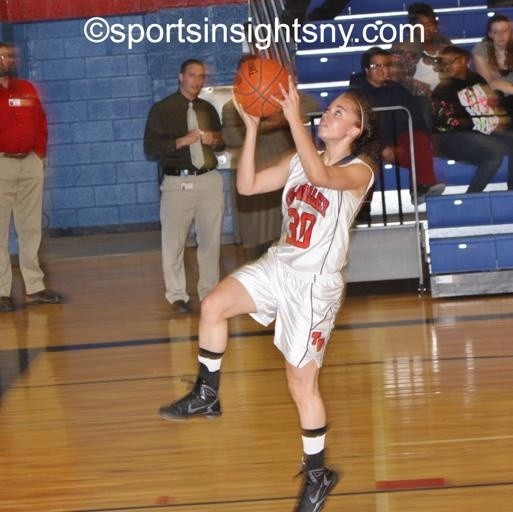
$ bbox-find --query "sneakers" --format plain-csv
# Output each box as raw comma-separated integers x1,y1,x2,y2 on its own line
157,376,223,424
0,296,16,313
409,182,446,207
171,299,190,315
24,288,66,305
292,455,340,512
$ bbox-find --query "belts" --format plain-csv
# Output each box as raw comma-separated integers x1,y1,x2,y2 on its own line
163,166,217,177
1,151,31,159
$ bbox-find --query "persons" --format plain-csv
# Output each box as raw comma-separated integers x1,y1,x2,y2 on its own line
216,52,321,263
278,1,349,36
139,57,230,316
156,69,375,510
0,39,64,311
342,3,512,227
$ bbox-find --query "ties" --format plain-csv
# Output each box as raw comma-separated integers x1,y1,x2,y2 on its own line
186,101,205,171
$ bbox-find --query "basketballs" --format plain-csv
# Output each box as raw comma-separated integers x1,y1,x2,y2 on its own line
234,58,289,117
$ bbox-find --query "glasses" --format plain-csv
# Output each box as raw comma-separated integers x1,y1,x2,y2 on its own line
440,56,463,68
368,63,390,70
0,55,10,60
